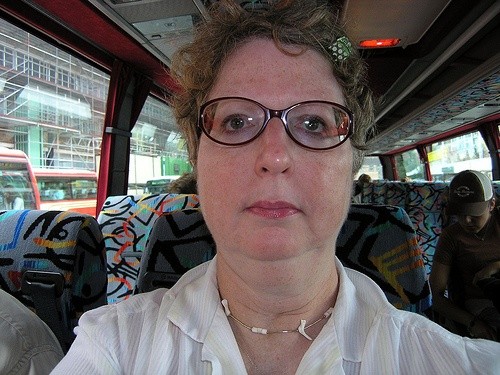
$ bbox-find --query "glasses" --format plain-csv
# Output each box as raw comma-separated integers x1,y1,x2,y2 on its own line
198,97,354,151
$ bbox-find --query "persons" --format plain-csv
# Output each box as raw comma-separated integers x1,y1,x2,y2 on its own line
43,2,500,375
169,173,197,195
352,174,370,203
0,289,63,375
431,170,500,344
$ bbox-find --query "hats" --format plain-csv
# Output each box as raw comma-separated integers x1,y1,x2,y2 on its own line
447,170,493,216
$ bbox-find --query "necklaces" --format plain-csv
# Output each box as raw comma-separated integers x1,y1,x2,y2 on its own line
221,299,334,341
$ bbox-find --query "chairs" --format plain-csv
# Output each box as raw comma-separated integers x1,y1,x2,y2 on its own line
0,178,500,357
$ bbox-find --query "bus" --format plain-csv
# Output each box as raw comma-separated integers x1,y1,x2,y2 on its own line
0,148,41,213
143,174,183,197
32,168,98,221
